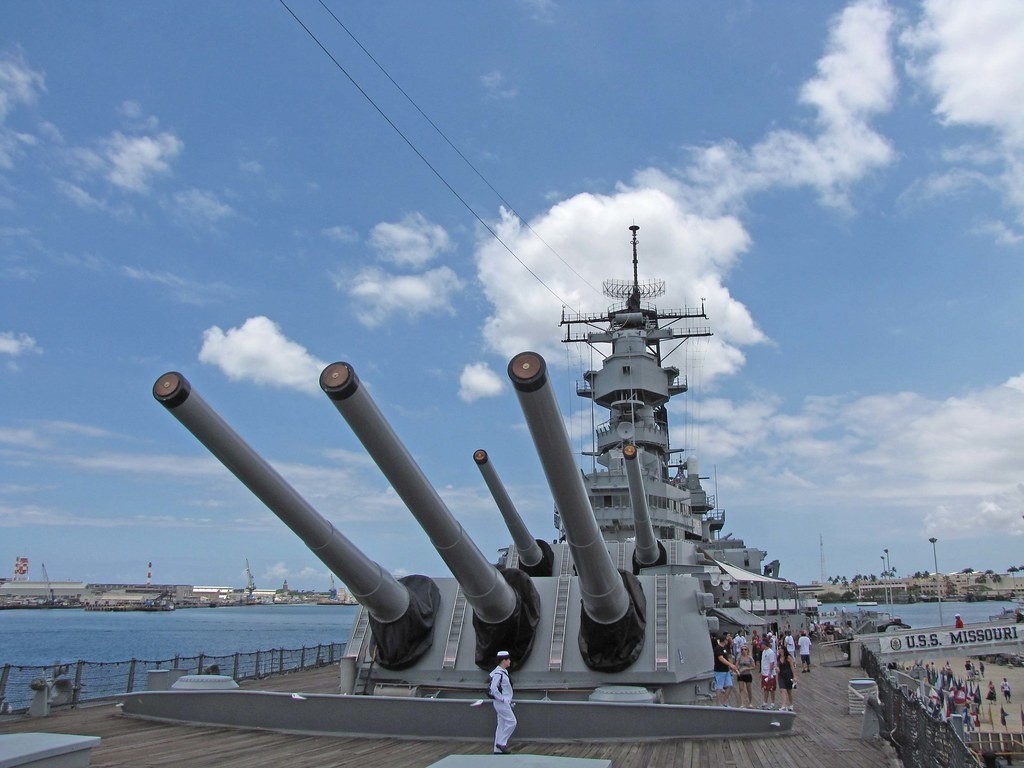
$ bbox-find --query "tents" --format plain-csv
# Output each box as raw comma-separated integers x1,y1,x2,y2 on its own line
707,607,765,636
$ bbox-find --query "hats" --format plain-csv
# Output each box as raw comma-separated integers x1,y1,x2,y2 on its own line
753,630,757,634
955,613,961,617
497,650,509,656
801,630,807,634
761,638,770,647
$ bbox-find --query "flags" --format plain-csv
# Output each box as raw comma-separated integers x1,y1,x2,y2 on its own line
911,675,1009,730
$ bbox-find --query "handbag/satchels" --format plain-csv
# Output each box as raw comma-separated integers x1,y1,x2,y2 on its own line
486,672,503,699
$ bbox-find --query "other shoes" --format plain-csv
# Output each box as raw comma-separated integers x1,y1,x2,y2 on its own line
1009,700,1011,703
802,670,806,673
993,703,996,705
794,665,796,669
788,705,794,711
740,705,746,707
756,704,770,709
767,705,776,710
778,706,786,711
1007,700,1009,703
806,669,810,672
749,704,753,707
991,702,993,704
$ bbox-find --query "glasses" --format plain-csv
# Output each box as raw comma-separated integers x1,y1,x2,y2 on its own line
741,648,748,651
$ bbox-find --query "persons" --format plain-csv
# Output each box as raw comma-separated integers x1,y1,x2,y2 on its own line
965,660,976,681
723,630,796,673
776,645,797,711
824,620,834,641
759,641,777,710
735,645,755,708
489,651,517,755
979,661,985,679
1014,608,1024,623
714,635,737,706
940,661,951,675
798,631,812,673
926,661,936,683
986,680,997,705
1000,678,1012,703
954,614,964,628
846,623,854,660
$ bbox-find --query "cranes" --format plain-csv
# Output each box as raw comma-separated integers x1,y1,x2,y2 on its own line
42,563,54,605
245,558,256,599
330,573,337,599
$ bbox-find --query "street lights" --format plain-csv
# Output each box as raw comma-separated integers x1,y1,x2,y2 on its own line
884,549,895,621
929,538,944,627
881,556,889,612
